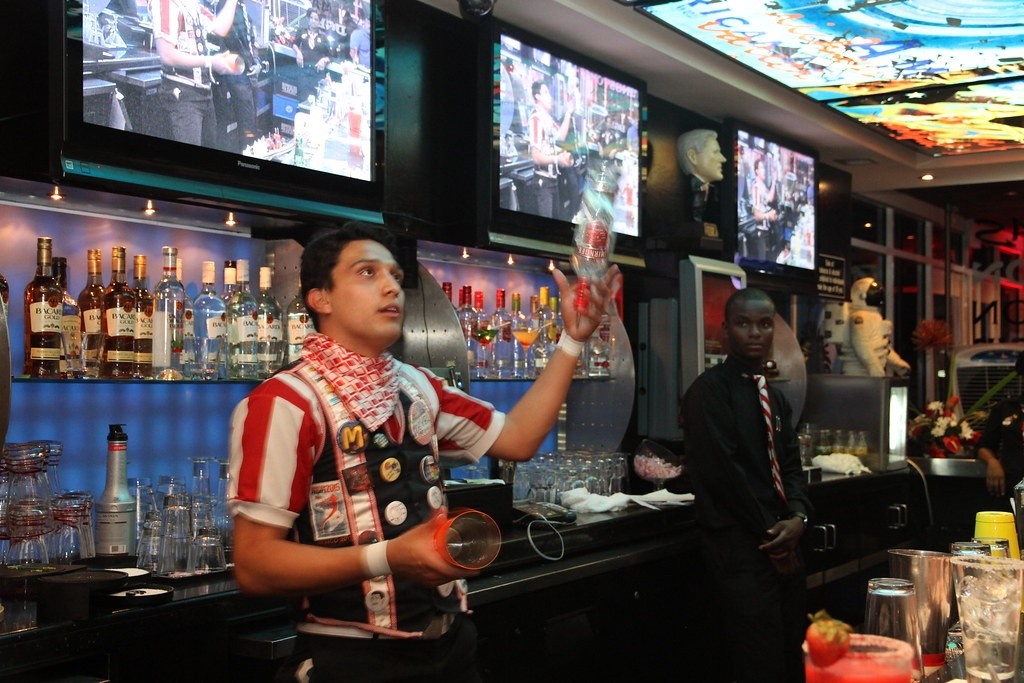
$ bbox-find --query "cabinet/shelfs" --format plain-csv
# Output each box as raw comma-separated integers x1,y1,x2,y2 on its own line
0,378,287,683
471,376,693,571
806,470,916,588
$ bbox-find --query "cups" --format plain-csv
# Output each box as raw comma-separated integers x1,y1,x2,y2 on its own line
972,537,1010,557
128,456,234,575
950,556,1024,683
887,548,953,667
433,511,502,570
514,449,626,504
801,634,915,683
60,331,105,379
1,440,96,571
862,578,925,683
951,543,990,556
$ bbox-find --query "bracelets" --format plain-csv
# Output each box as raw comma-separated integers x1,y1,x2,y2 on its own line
789,511,810,527
554,329,585,357
361,540,392,577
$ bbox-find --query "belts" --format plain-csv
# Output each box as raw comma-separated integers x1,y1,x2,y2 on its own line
757,226,769,230
160,70,212,90
533,168,558,179
297,614,457,639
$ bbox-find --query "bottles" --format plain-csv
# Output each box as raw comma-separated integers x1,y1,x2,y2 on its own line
815,429,833,456
78,246,153,380
797,422,815,467
23,237,60,378
572,180,619,311
95,423,137,564
832,429,867,466
443,281,612,377
0,273,8,321
153,246,318,380
51,258,81,378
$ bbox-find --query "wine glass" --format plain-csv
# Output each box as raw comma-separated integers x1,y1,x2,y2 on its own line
509,323,540,378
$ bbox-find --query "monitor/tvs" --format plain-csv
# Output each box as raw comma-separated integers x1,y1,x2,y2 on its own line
47,0,390,212
476,16,646,250
721,115,820,283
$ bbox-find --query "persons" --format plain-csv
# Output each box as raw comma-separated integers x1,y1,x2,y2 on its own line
972,351,1024,513
677,129,726,237
748,161,814,260
223,225,618,683
147,0,371,147
525,69,639,218
679,288,811,683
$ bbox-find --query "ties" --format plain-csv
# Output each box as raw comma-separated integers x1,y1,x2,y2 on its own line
740,374,787,508
572,117,579,153
237,2,253,60
776,182,782,211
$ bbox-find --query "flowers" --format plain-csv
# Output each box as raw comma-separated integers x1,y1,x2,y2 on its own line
907,320,1017,459
